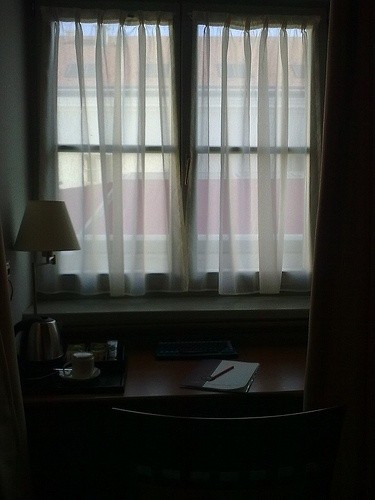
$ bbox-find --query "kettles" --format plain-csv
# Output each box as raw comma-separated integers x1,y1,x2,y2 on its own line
13,314,65,382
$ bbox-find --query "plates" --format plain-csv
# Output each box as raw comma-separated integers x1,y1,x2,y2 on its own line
58,366,101,383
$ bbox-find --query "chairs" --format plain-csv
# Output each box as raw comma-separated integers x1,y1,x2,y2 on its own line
110,404,346,499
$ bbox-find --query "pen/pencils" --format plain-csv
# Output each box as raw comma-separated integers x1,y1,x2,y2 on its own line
208,366,235,382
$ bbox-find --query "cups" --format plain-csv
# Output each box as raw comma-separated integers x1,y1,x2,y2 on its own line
71,351,95,378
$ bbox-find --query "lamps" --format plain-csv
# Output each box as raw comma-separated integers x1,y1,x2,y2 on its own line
9,200,82,322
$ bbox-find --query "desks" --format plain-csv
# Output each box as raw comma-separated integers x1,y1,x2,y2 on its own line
17,338,304,405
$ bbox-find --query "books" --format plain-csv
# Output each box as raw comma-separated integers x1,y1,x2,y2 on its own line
179,358,261,395
